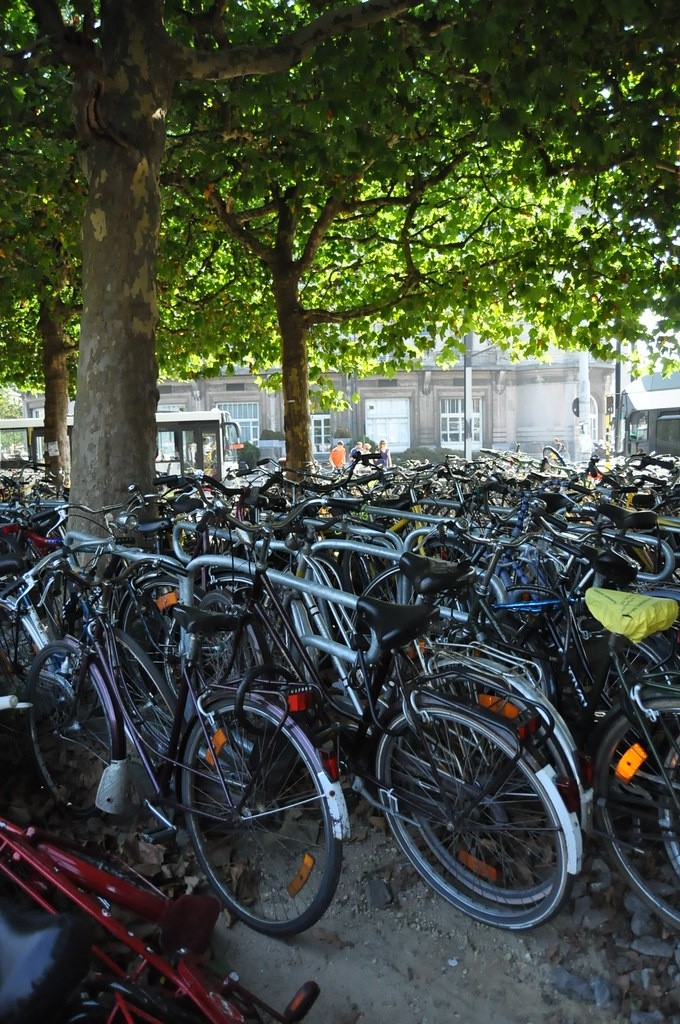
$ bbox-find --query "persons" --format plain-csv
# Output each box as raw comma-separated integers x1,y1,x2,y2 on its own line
329,441,346,470
348,442,363,466
362,444,374,465
553,438,563,454
375,440,392,467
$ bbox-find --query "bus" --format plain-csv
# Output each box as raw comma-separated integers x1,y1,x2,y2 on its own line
0,406,243,487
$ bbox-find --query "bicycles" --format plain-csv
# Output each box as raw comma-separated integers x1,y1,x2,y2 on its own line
1,689,320,1023
0,434,680,939
